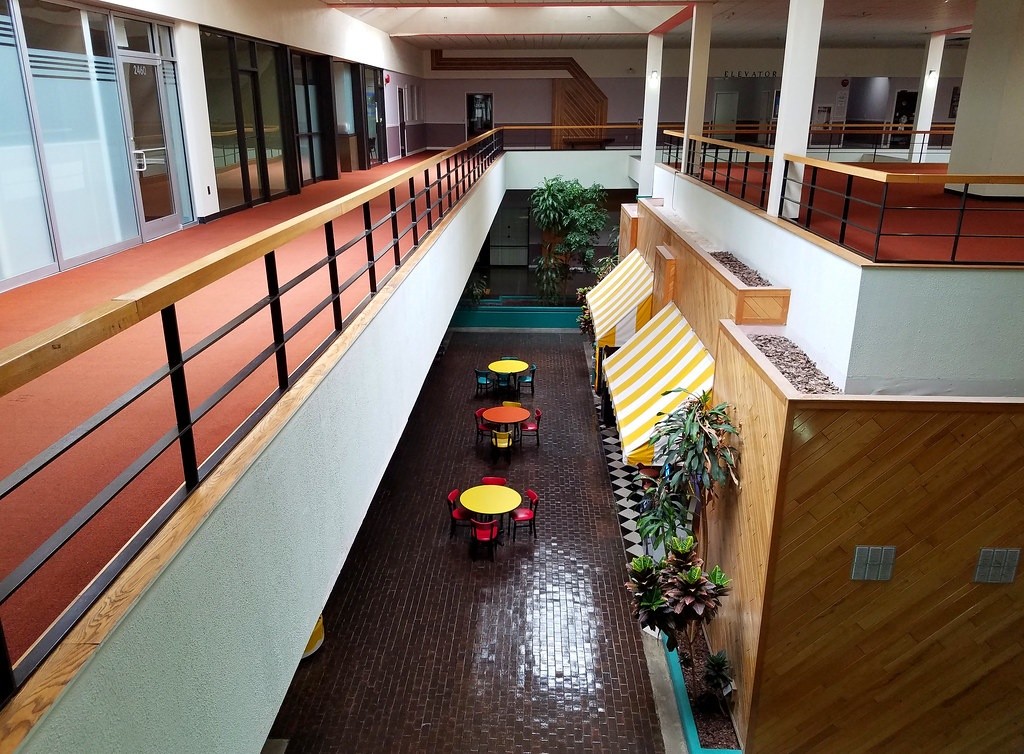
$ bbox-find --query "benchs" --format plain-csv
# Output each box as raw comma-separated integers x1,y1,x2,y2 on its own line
562,137,616,149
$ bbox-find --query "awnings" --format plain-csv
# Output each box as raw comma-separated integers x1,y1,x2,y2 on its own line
586,249,654,347
602,301,715,466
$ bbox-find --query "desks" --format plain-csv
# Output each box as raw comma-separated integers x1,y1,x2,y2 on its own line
488,359,529,391
482,407,530,447
459,484,522,545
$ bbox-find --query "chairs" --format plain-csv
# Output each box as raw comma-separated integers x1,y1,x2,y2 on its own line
475,407,501,442
517,364,537,397
501,401,521,434
490,429,514,465
474,368,492,398
447,488,476,539
469,518,499,562
507,489,539,542
481,477,507,487
500,357,519,381
494,372,512,397
518,409,542,448
